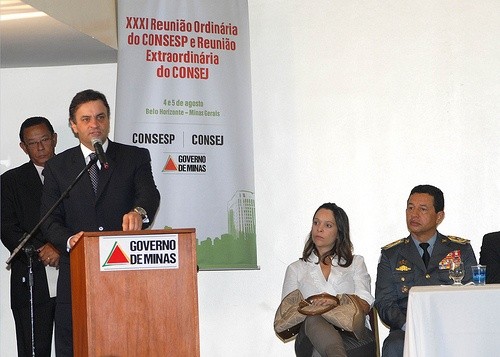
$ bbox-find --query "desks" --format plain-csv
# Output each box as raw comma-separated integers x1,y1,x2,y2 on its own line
402,284,500,357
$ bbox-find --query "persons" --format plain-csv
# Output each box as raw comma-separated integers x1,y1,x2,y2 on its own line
375,185,477,357
282,202,375,357
0,116,57,357
40,89,161,357
479,231,500,284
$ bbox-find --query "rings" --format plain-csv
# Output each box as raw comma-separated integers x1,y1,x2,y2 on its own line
48,257,51,260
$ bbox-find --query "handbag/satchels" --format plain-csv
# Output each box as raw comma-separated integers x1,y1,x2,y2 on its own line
274,289,366,343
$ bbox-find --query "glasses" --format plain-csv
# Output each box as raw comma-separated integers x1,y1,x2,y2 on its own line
25,137,53,147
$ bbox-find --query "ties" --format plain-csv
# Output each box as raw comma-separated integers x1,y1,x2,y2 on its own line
41,168,45,186
87,153,100,196
419,242,432,270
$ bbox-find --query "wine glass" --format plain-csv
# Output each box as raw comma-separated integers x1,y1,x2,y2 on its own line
449,261,465,285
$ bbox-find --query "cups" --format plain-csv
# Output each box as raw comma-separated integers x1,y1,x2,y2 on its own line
470,265,486,285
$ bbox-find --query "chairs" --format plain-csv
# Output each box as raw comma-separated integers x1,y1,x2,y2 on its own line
369,307,380,357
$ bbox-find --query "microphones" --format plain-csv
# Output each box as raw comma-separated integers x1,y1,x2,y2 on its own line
91,137,110,169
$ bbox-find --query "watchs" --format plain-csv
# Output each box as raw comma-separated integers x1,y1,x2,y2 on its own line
133,207,147,220
